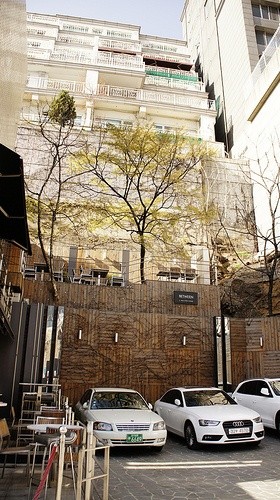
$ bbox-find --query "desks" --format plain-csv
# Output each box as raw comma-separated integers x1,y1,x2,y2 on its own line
26,424,83,500
157,271,198,281
90,269,109,287
33,262,49,281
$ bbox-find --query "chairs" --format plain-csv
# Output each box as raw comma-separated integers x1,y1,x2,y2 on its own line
0,393,84,489
168,266,200,281
22,258,127,287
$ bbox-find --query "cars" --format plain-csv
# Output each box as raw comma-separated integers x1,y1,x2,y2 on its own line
232,378,280,432
154,386,265,449
74,388,168,453
